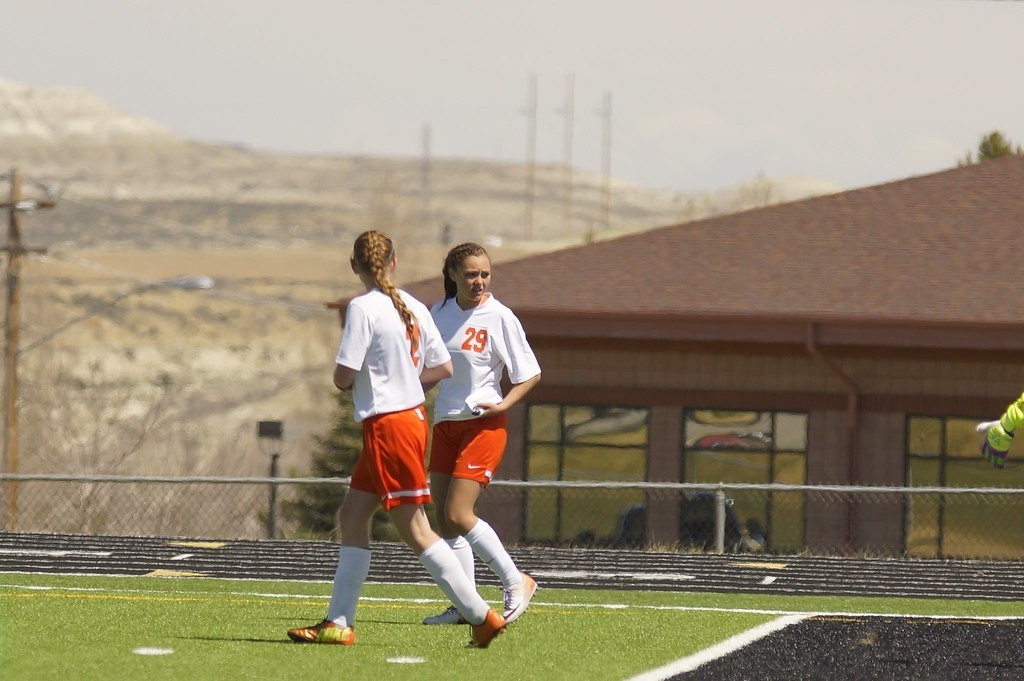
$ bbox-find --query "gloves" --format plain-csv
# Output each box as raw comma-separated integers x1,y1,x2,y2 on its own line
976,420,1013,470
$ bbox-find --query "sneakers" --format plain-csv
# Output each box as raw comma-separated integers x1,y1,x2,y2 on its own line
500,573,539,624
465,608,507,648
288,620,356,645
423,605,468,624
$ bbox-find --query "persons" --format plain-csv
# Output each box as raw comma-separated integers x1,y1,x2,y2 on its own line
402,241,541,626
975,393,1024,472
286,229,510,650
